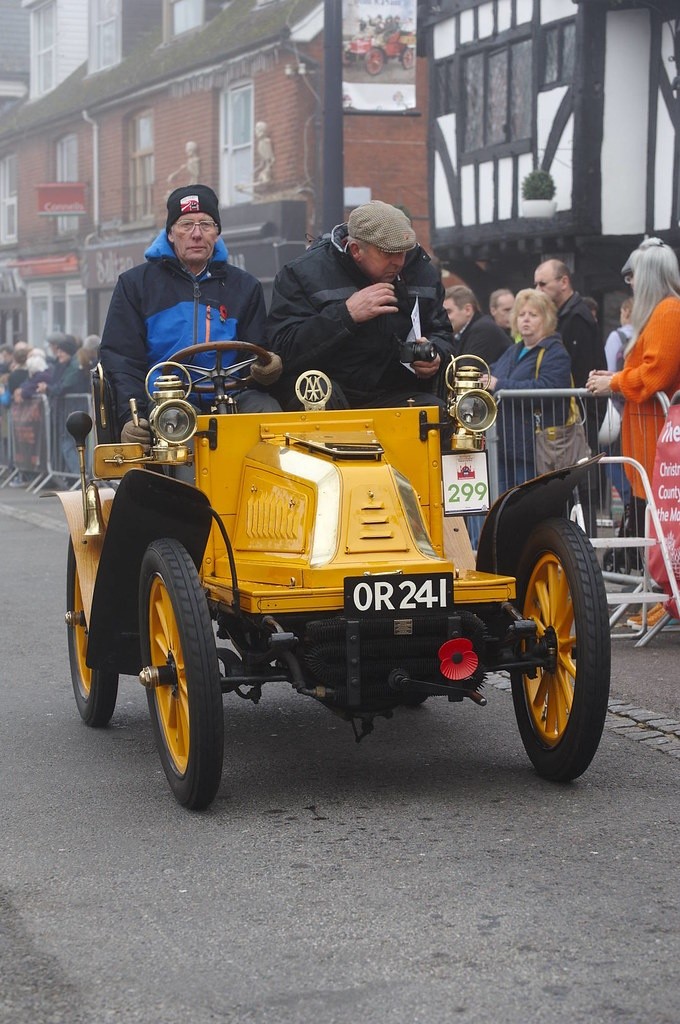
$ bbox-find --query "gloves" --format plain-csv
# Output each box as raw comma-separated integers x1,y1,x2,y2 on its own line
249,349,282,386
122,417,153,453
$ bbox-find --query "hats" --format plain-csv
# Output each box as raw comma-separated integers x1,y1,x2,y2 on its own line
59,335,78,355
165,183,222,236
347,200,416,252
46,332,67,345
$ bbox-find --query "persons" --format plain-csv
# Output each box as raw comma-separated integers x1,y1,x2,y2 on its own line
368,13,414,43
0,333,102,489
101,184,282,483
267,199,454,451
584,234,680,630
441,259,647,575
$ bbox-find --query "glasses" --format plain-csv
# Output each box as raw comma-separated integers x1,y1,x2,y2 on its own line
175,218,219,234
623,273,635,284
531,275,563,288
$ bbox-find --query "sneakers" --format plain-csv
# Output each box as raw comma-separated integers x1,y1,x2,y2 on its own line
627,603,680,632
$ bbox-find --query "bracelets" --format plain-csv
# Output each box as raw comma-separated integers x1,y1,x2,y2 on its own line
609,372,617,394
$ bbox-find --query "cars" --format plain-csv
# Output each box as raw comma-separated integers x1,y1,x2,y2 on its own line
57,341,611,811
342,31,415,76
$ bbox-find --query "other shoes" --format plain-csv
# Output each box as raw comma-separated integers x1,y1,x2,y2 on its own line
594,509,612,520
8,476,29,489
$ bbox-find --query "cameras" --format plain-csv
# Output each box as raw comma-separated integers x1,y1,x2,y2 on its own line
400,342,438,364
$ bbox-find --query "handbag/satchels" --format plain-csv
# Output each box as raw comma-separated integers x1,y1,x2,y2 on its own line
532,402,593,477
597,399,621,448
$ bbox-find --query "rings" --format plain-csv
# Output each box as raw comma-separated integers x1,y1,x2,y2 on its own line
593,380,595,384
593,385,595,390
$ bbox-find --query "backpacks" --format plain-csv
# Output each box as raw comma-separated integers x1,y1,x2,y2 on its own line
612,329,632,403
